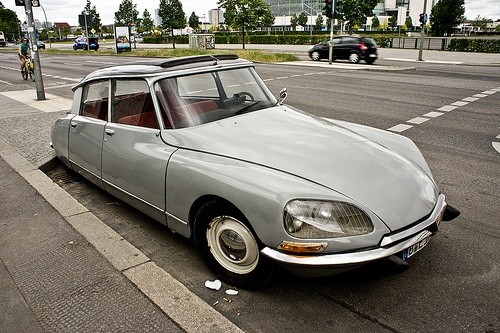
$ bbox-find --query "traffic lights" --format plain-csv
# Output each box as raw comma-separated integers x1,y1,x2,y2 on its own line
322,0,332,17
419,13,427,24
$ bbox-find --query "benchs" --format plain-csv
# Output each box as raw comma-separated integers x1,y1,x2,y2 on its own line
86,93,218,124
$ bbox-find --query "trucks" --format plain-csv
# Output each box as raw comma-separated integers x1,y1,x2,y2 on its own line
0,32,7,46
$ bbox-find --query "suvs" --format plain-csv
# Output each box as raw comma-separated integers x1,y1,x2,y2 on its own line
131,36,143,43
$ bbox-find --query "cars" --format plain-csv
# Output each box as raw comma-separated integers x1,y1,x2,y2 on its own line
73,37,99,51
36,41,45,50
49,55,447,288
308,36,378,64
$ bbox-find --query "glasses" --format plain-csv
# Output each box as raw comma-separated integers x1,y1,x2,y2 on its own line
24,40,27,41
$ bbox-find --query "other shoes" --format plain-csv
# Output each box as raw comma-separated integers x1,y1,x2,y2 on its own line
21,70,24,75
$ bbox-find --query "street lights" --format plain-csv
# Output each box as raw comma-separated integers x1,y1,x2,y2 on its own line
2,8,20,39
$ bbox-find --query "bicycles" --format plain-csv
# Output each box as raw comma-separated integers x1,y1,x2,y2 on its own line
20,56,35,82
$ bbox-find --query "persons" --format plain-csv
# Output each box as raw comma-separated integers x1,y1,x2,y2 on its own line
18,37,32,75
